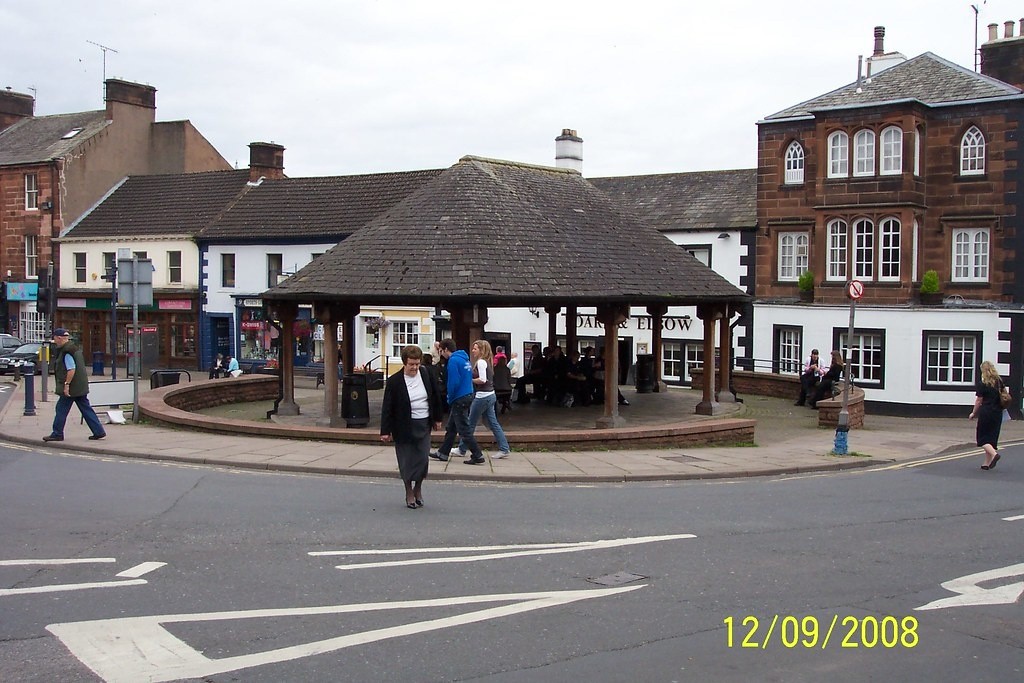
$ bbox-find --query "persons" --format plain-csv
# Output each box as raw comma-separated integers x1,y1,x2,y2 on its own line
421,342,449,414
209,353,239,380
794,349,825,406
807,350,844,410
337,343,343,383
449,340,512,459
380,345,443,509
493,346,508,367
513,344,630,408
42,328,107,441
507,353,519,384
429,338,485,464
493,356,518,414
968,360,1007,470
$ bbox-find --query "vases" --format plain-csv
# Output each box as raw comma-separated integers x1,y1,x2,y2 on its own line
373,323,381,328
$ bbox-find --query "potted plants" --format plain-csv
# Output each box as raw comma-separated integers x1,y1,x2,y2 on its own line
798,271,814,303
920,269,944,305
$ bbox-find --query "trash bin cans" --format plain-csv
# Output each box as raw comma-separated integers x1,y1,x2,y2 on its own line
636,353,656,393
151,370,181,390
339,372,370,429
92,351,105,376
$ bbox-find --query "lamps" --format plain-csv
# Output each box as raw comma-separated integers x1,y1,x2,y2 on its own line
42,201,49,210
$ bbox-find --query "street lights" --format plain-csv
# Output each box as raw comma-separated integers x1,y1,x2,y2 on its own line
102,274,117,381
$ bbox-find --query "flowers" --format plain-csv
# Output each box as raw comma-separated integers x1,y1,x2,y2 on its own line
366,316,392,327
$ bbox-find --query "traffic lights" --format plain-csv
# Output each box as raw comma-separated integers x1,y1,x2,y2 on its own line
37,287,48,312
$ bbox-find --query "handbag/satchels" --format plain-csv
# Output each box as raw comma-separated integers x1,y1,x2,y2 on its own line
224,370,231,377
562,392,576,408
831,380,852,397
997,376,1012,409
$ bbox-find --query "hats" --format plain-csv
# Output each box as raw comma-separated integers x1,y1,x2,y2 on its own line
217,353,223,358
50,328,70,336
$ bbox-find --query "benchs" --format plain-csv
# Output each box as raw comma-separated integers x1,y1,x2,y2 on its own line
210,366,242,375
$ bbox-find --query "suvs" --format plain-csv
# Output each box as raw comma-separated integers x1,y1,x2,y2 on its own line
0,334,25,354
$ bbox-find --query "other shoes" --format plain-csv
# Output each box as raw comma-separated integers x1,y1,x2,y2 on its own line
451,447,465,457
981,466,989,470
429,450,448,461
43,435,64,441
794,402,805,406
89,432,106,440
491,450,510,459
405,497,417,509
412,487,424,506
989,454,1000,469
463,454,485,465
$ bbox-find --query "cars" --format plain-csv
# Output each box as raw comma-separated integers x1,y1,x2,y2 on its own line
0,342,56,375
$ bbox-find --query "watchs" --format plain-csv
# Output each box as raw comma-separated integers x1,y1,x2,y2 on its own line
64,382,70,385
